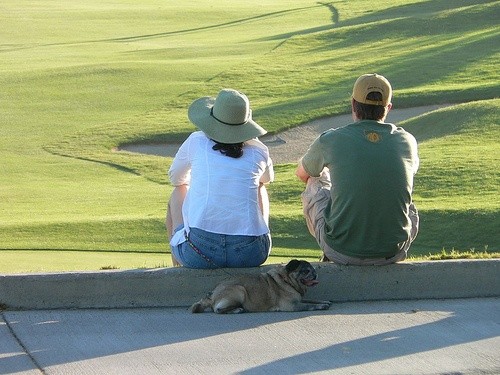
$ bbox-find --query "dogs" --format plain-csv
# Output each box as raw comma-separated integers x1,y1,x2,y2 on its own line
192,258,332,314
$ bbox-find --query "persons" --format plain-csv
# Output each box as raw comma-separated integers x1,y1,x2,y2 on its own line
165,89,273,269
296,73,419,265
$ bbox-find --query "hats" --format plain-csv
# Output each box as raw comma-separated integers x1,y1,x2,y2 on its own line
352,74,393,106
188,88,267,145
